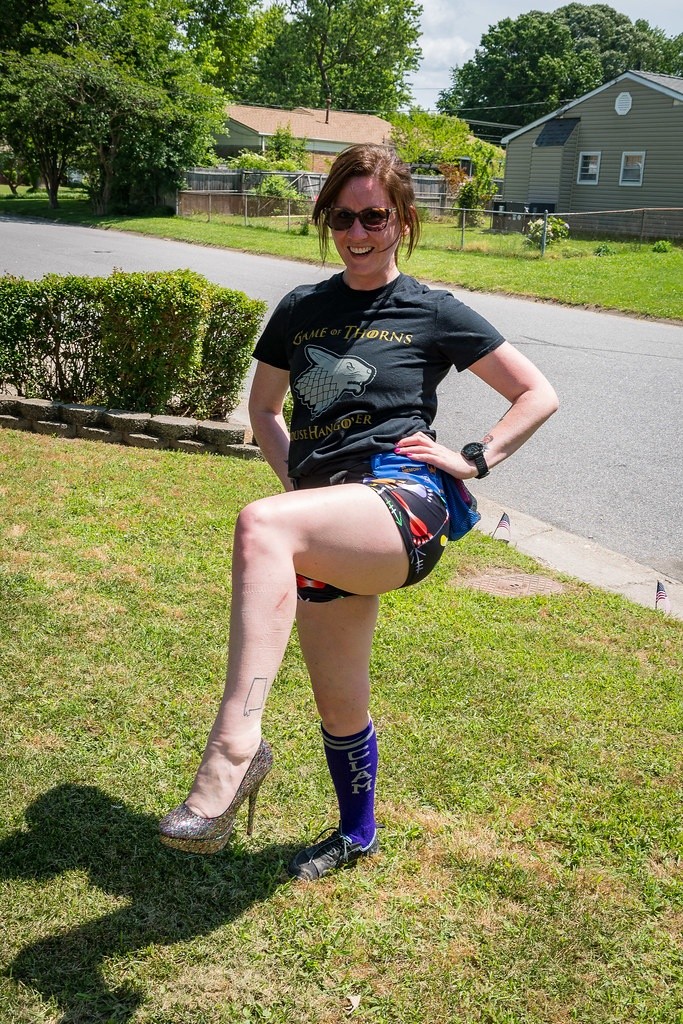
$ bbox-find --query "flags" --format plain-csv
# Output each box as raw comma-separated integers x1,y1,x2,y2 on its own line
496,512,510,532
656,582,667,603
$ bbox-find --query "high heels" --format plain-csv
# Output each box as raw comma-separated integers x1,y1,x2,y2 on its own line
159,740,273,855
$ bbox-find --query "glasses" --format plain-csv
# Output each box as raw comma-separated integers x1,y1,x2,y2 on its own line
321,206,398,232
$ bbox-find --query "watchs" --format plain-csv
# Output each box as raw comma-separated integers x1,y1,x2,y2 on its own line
461,442,490,479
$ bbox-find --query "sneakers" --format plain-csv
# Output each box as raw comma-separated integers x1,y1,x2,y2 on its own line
285,820,386,882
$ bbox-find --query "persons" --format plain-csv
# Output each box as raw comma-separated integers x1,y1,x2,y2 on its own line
157,142,559,883
